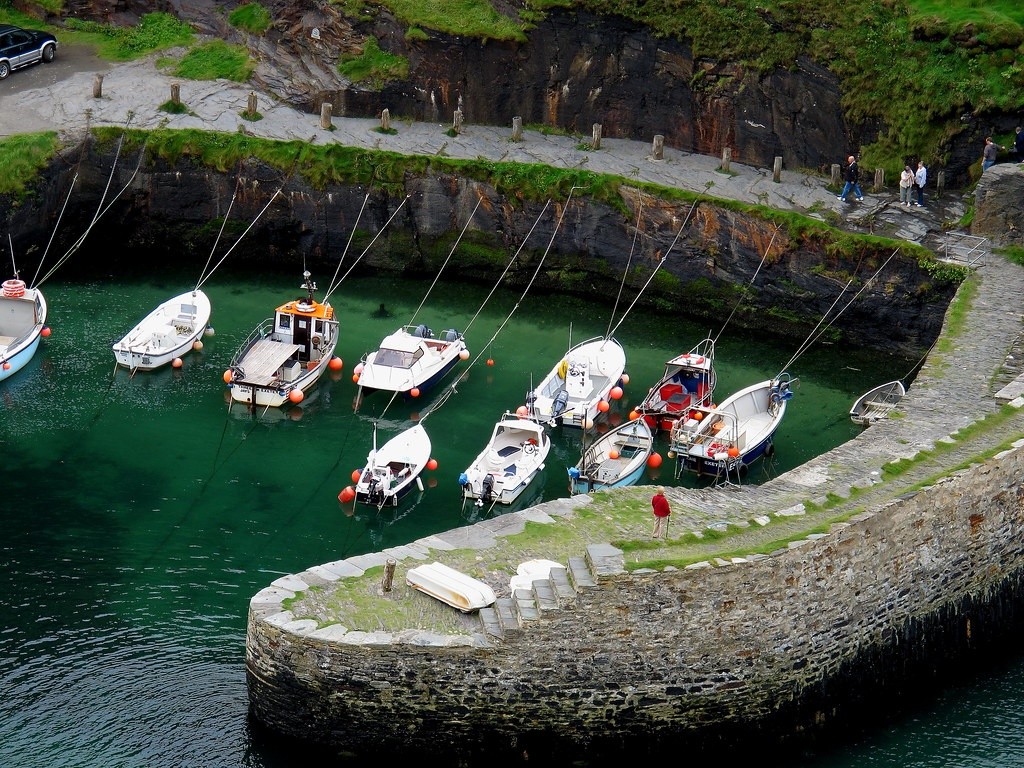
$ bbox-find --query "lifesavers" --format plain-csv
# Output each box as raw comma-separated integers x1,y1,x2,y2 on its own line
296,308,316,313
682,353,705,365
558,360,568,379
295,299,317,309
2,279,25,297
708,444,726,457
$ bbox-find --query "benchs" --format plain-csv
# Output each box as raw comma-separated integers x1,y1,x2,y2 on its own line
864,401,894,408
615,441,648,449
497,445,521,458
171,318,196,325
652,401,667,411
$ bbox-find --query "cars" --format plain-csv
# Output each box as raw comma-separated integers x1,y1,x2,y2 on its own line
0,24,59,80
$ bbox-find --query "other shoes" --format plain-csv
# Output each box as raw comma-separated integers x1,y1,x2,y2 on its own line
914,202,923,206
900,201,911,207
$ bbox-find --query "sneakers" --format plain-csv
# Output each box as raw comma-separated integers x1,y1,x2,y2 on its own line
856,197,863,201
836,197,845,201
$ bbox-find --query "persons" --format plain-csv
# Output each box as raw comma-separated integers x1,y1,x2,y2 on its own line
899,161,926,207
652,486,671,538
981,137,1005,173
837,156,863,201
1014,127,1024,163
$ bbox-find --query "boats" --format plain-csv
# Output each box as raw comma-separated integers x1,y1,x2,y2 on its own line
0,233,47,383
635,337,718,432
511,559,567,599
849,380,905,428
354,422,432,510
525,321,626,429
356,324,467,402
669,374,801,478
228,252,340,407
406,561,497,613
458,372,552,507
569,408,654,496
112,289,212,373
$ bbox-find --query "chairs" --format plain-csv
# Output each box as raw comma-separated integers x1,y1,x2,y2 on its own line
486,448,504,464
